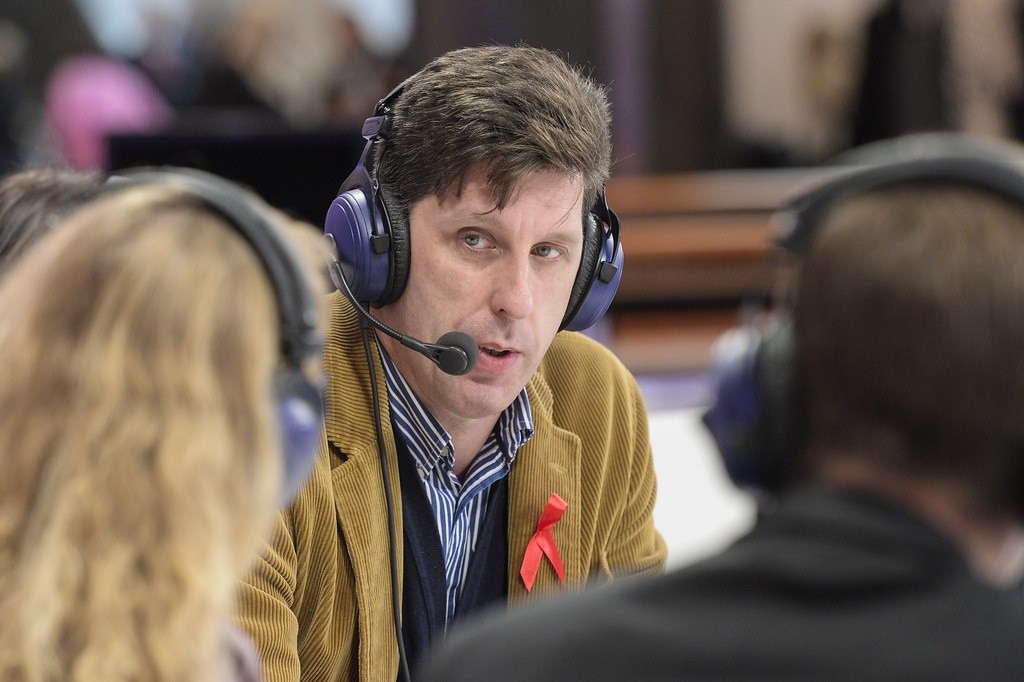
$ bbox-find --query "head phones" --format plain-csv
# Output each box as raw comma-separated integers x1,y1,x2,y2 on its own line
321,67,624,334
700,132,1024,499
35,162,324,511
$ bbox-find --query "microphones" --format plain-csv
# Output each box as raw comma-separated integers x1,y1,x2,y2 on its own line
321,232,479,375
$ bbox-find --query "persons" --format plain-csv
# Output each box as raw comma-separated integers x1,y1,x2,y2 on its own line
0,0,177,174
233,44,668,682
421,133,1024,682
0,165,335,682
